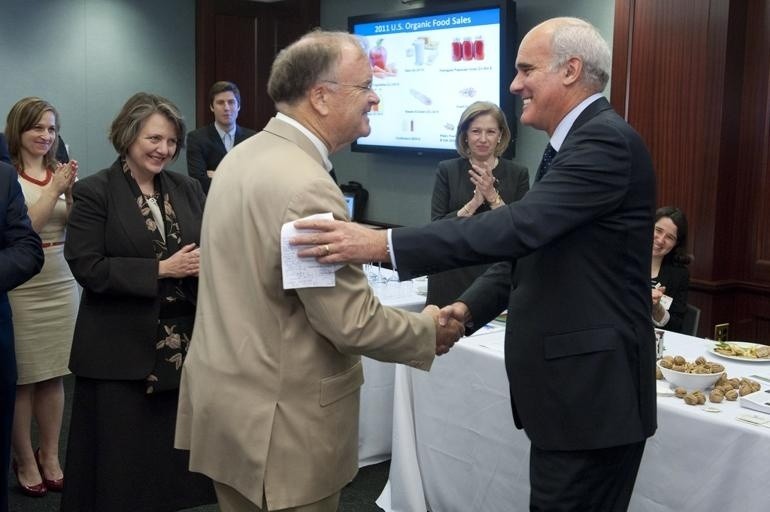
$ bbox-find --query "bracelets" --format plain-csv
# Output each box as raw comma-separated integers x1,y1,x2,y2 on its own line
463,203,474,216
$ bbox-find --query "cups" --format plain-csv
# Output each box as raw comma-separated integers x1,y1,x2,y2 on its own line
708,342,770,362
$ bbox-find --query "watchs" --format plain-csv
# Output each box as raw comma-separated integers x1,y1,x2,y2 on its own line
487,194,501,206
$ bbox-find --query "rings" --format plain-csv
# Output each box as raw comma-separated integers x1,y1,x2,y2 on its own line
325,244,331,256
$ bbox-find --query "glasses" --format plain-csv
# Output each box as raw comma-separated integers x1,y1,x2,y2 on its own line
318,75,376,94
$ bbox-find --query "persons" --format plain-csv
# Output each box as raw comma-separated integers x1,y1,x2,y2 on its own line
291,16,659,511
1,158,44,512
425,102,531,307
184,80,258,198
174,28,467,512
5,97,85,498
646,204,691,331
56,92,204,512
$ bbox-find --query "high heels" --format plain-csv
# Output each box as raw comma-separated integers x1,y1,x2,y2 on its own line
12,454,45,495
35,444,65,492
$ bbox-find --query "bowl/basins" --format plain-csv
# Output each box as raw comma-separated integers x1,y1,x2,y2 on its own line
657,359,726,390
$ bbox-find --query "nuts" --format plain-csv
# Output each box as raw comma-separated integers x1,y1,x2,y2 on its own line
652,347,760,404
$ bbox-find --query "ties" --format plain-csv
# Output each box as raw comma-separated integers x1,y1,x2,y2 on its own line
536,141,554,178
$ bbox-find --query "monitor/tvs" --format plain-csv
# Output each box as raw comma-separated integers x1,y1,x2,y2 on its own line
348,1,517,162
